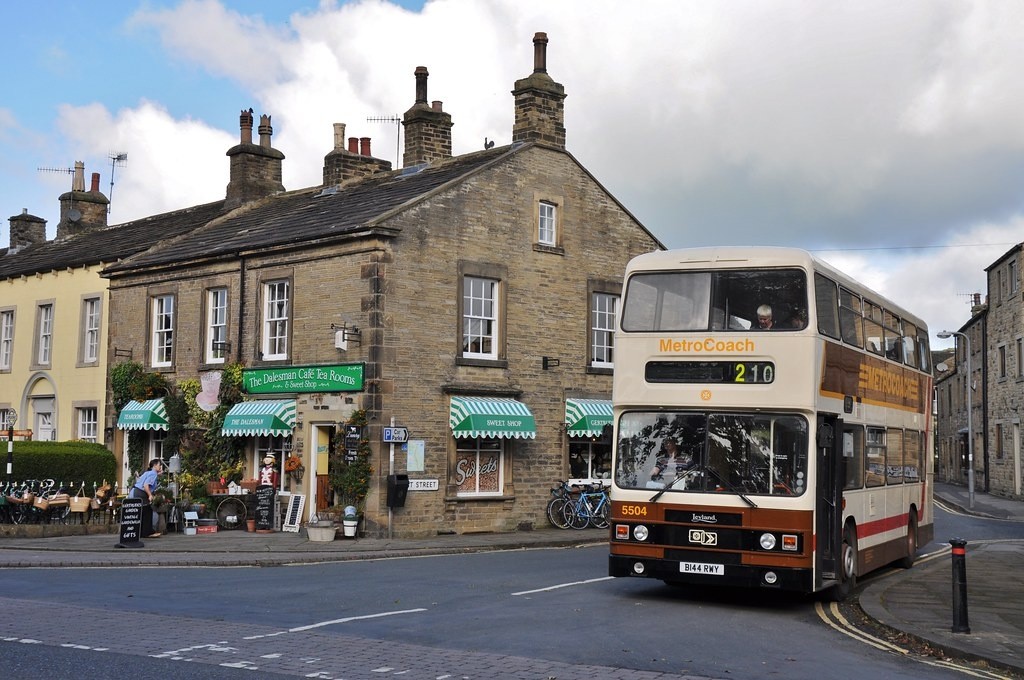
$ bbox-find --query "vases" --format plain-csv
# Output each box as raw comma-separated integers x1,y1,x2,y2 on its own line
306,525,338,542
342,516,360,537
246,519,255,533
209,482,232,496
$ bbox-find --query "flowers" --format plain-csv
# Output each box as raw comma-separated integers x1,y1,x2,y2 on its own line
332,406,375,506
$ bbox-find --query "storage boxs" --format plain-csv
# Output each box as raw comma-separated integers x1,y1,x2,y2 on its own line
184,526,218,535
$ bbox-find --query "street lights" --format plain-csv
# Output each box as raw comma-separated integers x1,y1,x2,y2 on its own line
936,330,974,509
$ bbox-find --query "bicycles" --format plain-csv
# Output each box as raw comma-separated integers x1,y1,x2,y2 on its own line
546,479,610,530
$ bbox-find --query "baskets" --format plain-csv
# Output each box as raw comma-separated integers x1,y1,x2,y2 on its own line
90,490,100,509
69,487,91,512
5,491,23,504
102,479,111,491
49,490,69,505
22,490,33,504
33,492,49,510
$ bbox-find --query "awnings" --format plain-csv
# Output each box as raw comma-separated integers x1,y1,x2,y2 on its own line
449,395,536,440
222,399,296,438
565,398,614,438
117,396,170,432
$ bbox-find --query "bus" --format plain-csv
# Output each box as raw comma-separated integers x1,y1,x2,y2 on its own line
608,246,937,595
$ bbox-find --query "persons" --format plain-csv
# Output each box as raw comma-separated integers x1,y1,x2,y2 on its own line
750,305,780,330
128,459,164,538
648,434,696,489
791,313,807,328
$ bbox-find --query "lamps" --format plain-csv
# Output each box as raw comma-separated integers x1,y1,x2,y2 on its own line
296,412,303,428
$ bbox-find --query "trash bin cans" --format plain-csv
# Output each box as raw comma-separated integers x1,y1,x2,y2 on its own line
386,475,410,507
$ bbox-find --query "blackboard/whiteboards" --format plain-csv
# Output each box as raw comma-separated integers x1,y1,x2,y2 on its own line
255,485,274,529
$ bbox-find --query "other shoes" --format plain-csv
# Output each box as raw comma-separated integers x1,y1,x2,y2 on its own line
149,532,161,537
